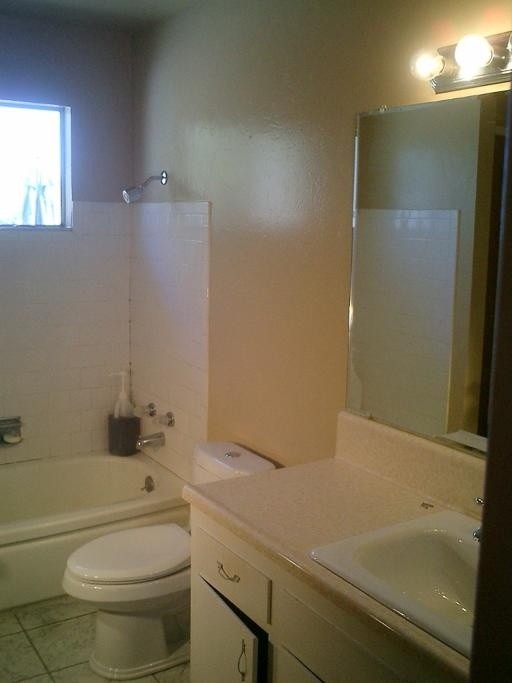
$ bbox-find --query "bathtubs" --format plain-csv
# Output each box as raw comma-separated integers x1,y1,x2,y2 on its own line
0,450,196,611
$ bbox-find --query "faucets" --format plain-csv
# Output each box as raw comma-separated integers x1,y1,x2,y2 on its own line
134,433,169,450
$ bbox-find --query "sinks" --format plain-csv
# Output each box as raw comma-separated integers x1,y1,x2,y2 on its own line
310,509,482,660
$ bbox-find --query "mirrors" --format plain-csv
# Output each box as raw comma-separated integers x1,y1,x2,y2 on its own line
345,90,508,461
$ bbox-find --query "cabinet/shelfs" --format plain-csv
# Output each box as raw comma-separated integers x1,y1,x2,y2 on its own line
190,503,467,681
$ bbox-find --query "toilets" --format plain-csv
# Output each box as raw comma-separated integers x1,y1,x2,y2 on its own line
61,438,277,682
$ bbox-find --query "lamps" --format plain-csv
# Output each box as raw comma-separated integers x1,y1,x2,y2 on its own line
408,44,453,89
454,33,502,74
